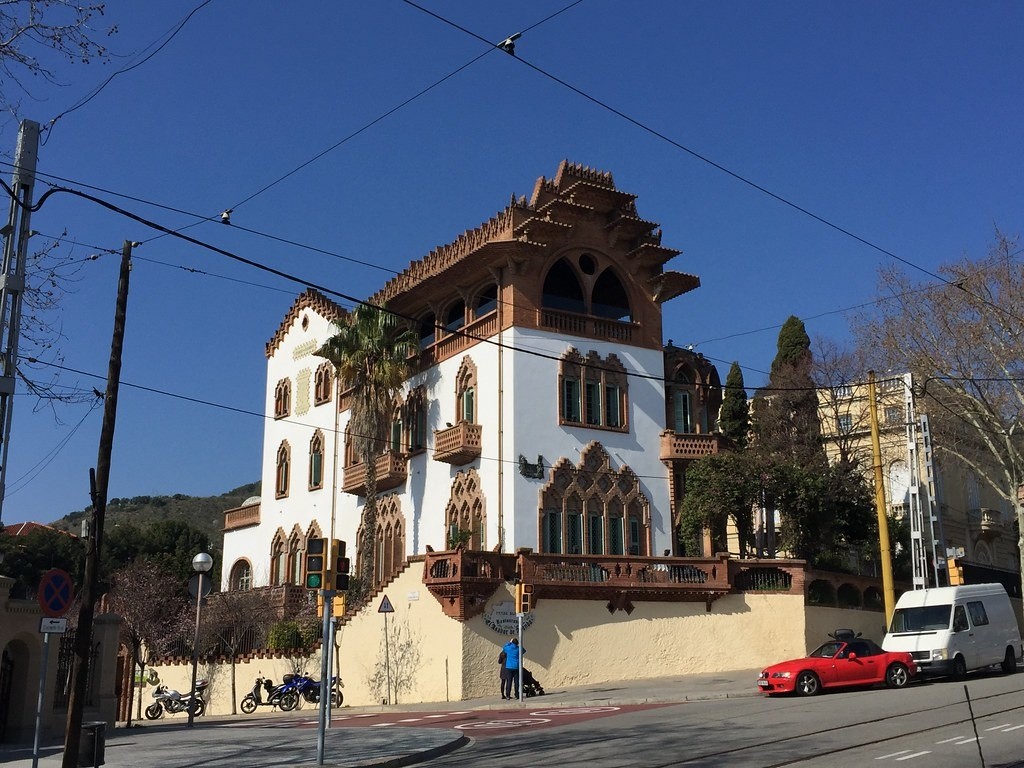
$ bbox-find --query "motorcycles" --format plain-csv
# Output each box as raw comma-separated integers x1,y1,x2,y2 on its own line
144,678,209,720
242,672,299,713
278,670,344,713
828,628,864,644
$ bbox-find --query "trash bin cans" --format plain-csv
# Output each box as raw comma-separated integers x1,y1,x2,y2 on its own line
78,721,108,768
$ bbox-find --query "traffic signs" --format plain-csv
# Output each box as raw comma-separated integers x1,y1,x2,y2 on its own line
335,540,353,593
306,536,326,590
39,617,68,633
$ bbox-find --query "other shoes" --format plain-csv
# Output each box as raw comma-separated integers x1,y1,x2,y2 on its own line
502,696,519,700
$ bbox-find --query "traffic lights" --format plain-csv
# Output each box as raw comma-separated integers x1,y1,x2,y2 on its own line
521,583,534,614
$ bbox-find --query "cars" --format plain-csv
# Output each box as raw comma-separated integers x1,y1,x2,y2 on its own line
756,637,919,696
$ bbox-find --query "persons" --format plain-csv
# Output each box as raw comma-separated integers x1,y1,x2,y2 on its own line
498,638,526,700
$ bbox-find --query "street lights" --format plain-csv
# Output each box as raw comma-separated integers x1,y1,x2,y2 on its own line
186,550,214,727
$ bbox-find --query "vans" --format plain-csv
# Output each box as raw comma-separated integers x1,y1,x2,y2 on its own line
880,582,1024,681
